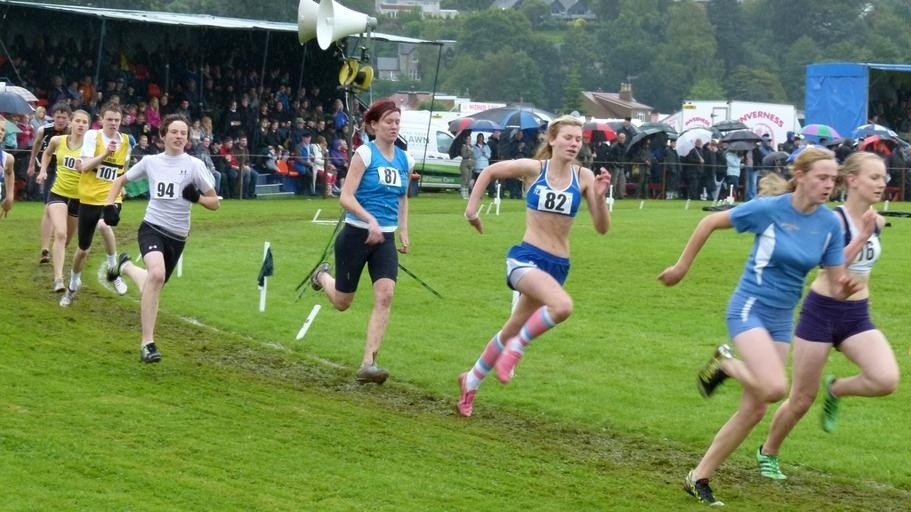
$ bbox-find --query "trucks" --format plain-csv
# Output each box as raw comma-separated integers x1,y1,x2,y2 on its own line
387,102,508,163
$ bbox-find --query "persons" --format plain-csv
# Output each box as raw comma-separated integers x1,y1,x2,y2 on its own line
0,115,16,220
755,150,900,484
25,103,73,264
658,143,862,503
455,115,612,420
0,25,368,200
307,101,412,387
103,113,224,366
35,108,93,293
55,99,130,309
449,134,911,202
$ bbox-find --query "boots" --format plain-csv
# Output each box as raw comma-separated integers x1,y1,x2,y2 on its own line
460,187,469,200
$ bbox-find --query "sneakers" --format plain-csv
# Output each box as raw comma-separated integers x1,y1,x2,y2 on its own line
756,445,789,480
55,277,82,307
821,375,839,433
356,352,389,381
40,249,50,263
140,343,162,362
683,471,725,507
311,262,328,291
697,344,737,399
457,372,477,417
107,254,132,295
495,338,521,383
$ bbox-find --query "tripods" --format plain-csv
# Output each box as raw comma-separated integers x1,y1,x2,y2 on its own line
296,208,444,303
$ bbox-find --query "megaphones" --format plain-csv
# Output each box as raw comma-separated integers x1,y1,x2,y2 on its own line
298,0,319,44
316,0,377,51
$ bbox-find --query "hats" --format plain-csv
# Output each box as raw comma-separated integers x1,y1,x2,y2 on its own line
363,100,395,120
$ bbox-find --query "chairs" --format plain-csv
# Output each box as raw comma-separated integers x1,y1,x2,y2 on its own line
274,158,299,194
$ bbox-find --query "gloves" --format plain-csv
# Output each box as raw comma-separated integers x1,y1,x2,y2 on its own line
104,205,119,226
183,184,200,202
